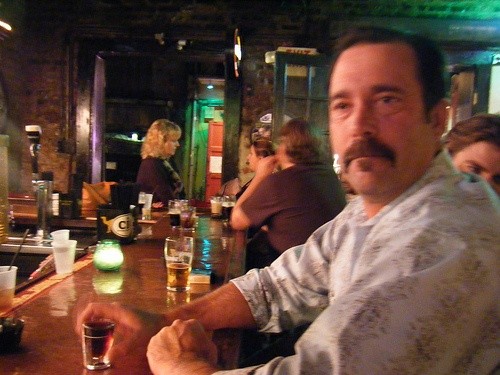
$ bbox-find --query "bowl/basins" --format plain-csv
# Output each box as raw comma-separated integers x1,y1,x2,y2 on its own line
0,317,24,354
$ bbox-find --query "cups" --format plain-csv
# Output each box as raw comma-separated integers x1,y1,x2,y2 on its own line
164,236,194,291
168,199,199,231
82,320,113,370
210,195,236,220
95,204,135,244
0,266,18,310
50,230,78,274
138,192,152,220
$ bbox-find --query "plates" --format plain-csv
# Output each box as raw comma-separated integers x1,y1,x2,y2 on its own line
152,207,164,211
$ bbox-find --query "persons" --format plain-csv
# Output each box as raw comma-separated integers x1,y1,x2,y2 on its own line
135,120,186,214
74,26,500,375
226,118,347,271
443,114,500,195
